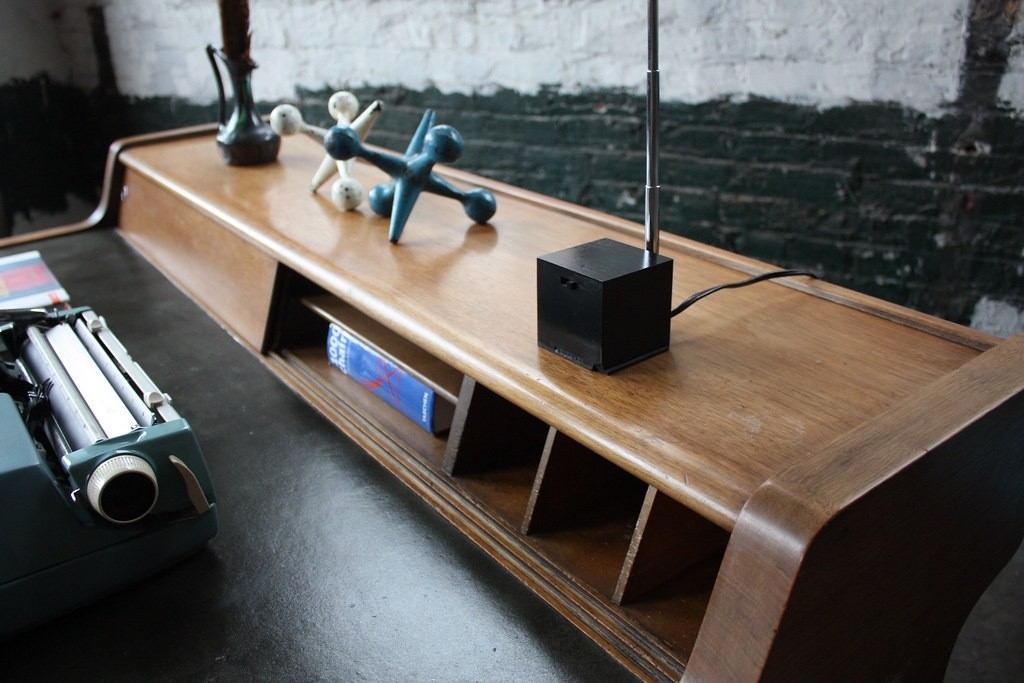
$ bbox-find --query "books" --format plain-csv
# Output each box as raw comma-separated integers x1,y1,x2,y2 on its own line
326,323,456,437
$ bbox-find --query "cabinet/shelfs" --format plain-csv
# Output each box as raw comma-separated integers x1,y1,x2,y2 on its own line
112,116,1024,683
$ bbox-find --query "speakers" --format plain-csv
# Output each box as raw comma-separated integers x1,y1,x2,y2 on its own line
536,238,674,375
